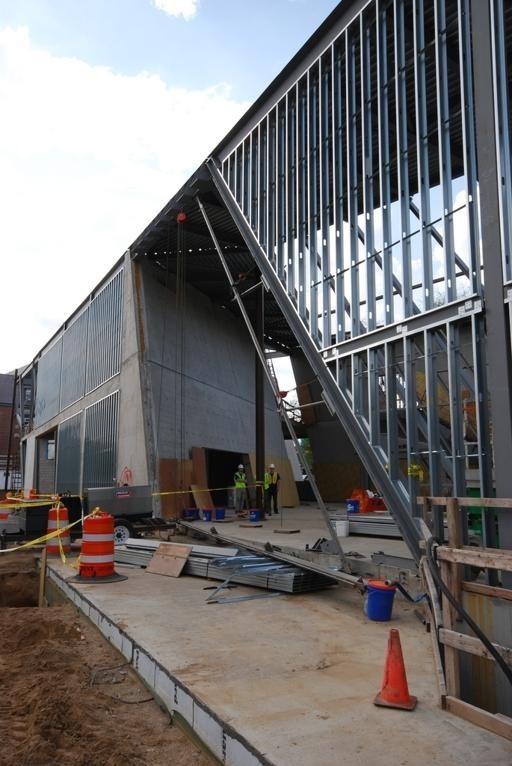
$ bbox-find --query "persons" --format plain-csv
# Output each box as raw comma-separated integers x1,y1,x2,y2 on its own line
234,464,247,513
264,464,280,515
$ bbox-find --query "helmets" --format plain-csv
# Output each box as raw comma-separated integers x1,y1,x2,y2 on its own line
269,464,275,467
238,464,244,469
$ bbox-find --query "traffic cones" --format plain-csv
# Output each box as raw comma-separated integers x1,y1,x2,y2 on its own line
374,625,418,711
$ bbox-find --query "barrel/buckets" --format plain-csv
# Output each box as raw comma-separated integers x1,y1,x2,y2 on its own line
216,507,225,520
202,508,212,520
367,580,397,623
249,509,260,522
346,498,360,515
337,520,350,537
183,507,200,520
